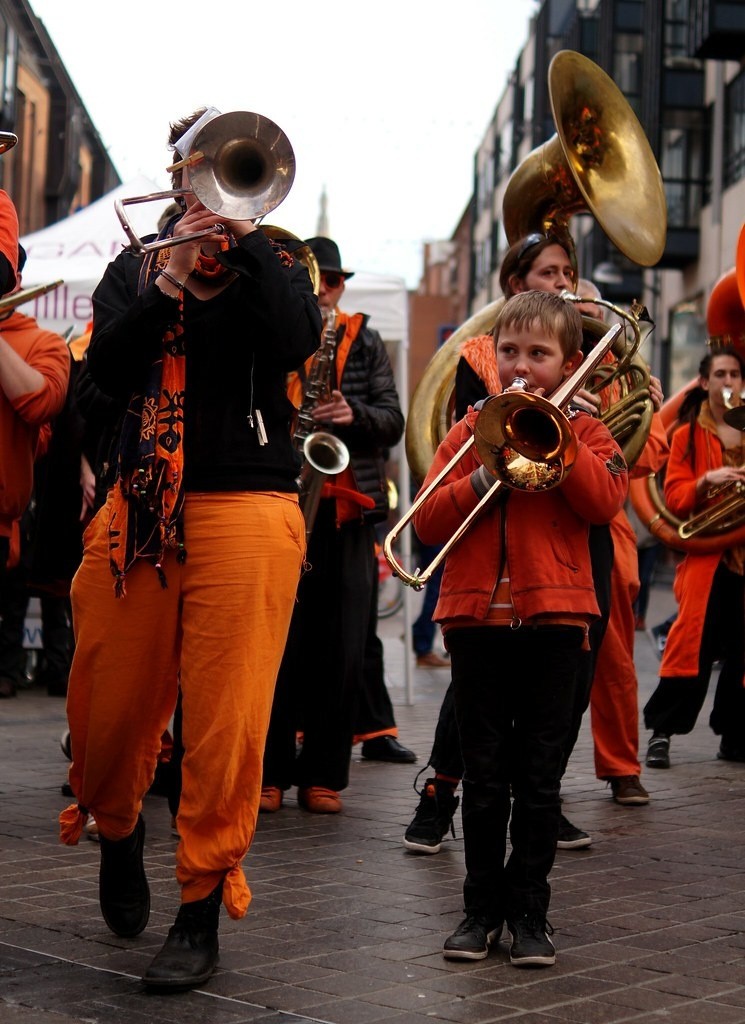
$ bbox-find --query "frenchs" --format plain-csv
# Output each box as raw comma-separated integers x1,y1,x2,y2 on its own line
401,46,671,510
645,330,745,541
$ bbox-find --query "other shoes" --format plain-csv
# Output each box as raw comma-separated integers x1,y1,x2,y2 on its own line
86,814,101,840
0,682,17,697
646,626,667,661
170,816,180,835
417,652,450,668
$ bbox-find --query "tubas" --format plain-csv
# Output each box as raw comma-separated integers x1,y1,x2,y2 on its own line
256,222,323,302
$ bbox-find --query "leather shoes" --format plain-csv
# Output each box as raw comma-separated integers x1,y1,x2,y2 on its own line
362,734,417,763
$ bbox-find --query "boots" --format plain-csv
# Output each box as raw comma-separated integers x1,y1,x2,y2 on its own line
139,879,224,989
98,813,150,935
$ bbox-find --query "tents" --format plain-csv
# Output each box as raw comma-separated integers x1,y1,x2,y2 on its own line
18,176,176,336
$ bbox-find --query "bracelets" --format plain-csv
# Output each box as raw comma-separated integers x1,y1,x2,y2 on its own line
160,270,184,291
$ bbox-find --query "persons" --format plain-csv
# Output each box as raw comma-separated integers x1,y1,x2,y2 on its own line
59,111,321,993
61,236,417,833
1,190,93,701
401,235,669,854
644,344,745,768
412,292,627,965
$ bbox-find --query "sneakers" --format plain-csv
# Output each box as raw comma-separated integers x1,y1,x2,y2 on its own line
257,786,283,812
297,783,342,813
646,737,670,768
556,813,591,848
445,916,504,960
505,919,555,965
716,741,745,760
608,775,649,805
402,777,459,853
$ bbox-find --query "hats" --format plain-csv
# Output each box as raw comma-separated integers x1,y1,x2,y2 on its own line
304,236,354,280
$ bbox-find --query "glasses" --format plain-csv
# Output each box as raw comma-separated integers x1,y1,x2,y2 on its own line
320,275,342,288
512,233,546,271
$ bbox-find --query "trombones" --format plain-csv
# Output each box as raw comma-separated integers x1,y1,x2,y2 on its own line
112,109,298,257
381,318,624,594
0,130,20,159
0,277,65,318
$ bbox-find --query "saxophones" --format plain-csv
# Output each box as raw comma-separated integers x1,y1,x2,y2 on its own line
286,303,353,576
